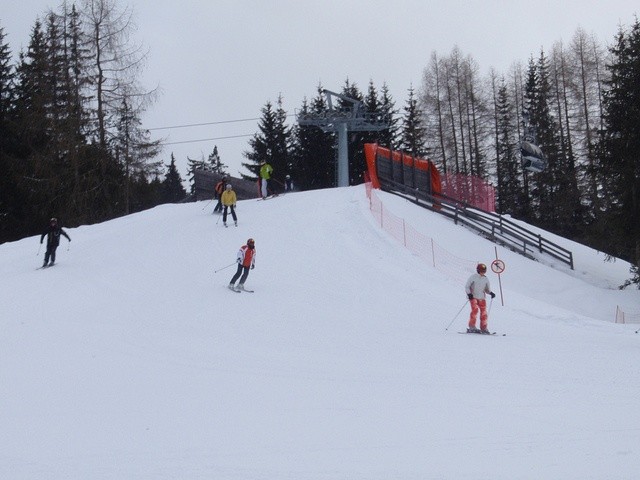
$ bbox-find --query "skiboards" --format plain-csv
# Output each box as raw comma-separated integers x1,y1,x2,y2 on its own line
223,222,238,228
458,331,506,337
228,286,255,294
36,262,57,270
257,194,279,201
213,212,232,215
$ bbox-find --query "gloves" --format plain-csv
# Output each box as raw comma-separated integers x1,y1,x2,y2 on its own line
468,294,473,299
251,265,254,270
236,258,240,264
490,292,496,298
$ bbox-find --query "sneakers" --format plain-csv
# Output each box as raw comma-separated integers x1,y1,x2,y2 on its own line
467,326,480,333
43,262,47,267
238,285,244,289
481,329,490,334
49,263,53,266
229,284,234,289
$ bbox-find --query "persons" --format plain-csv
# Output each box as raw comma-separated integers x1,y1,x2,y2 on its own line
214,176,228,214
466,263,495,333
260,161,272,199
40,218,71,267
222,185,238,223
229,238,256,288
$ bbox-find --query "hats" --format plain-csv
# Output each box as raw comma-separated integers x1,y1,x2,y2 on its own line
260,159,265,164
50,217,57,220
226,183,232,189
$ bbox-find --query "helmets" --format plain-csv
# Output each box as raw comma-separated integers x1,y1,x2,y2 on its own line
246,238,254,248
478,264,486,275
221,174,230,181
